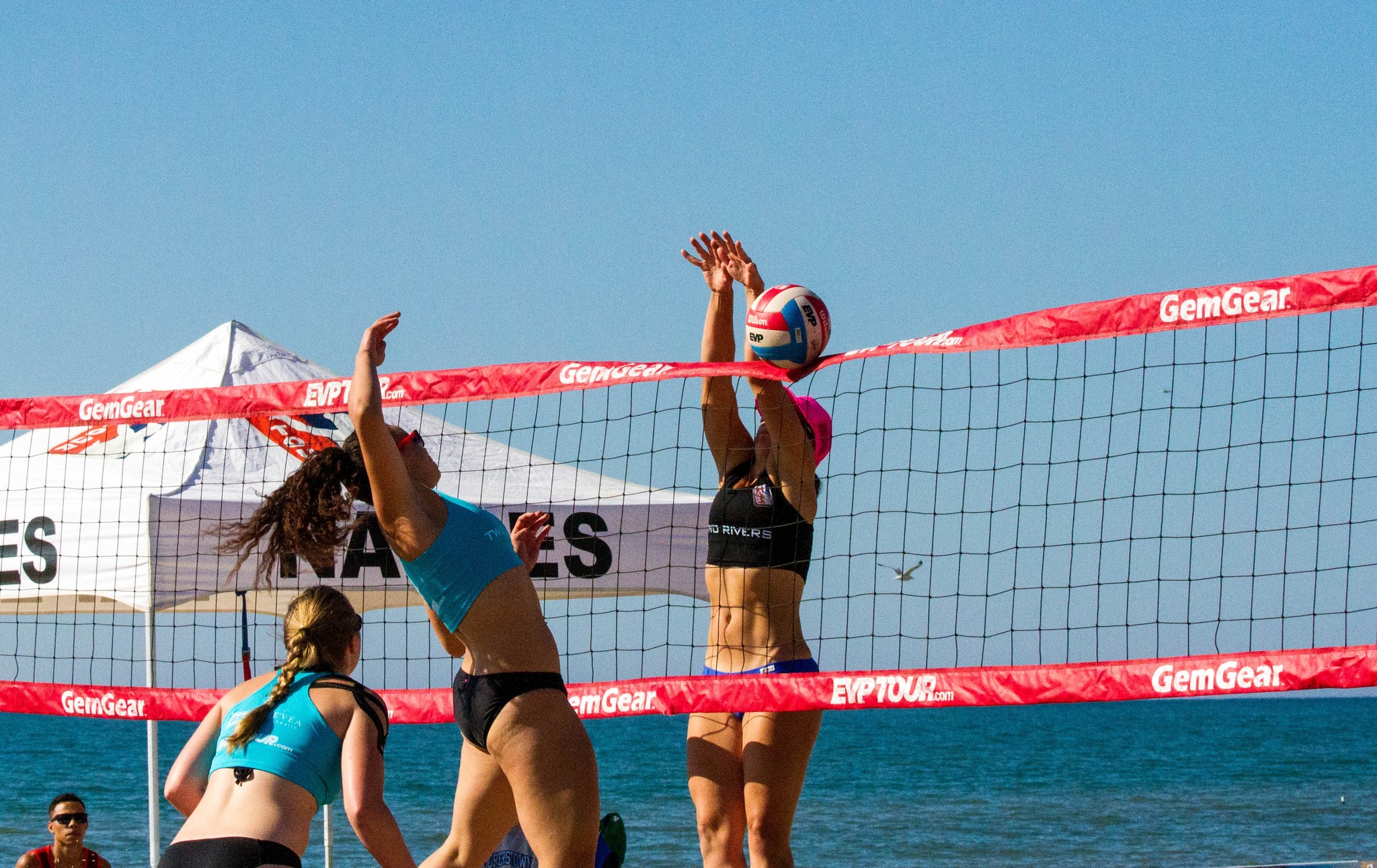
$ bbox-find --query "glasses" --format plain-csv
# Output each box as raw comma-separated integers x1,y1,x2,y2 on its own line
397,428,425,450
352,614,362,636
51,812,88,824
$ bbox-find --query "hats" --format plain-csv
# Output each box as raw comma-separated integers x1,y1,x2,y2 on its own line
783,385,833,466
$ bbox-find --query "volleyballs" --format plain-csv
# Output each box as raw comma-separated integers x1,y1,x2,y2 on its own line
745,284,831,370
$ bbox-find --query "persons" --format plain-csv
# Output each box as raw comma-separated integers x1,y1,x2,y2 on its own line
680,226,831,868
145,582,413,868
14,793,112,868
332,310,603,868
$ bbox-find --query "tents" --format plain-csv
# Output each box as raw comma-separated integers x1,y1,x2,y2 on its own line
0,320,730,868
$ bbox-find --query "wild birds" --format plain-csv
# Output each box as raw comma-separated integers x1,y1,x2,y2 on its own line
878,560,923,581
